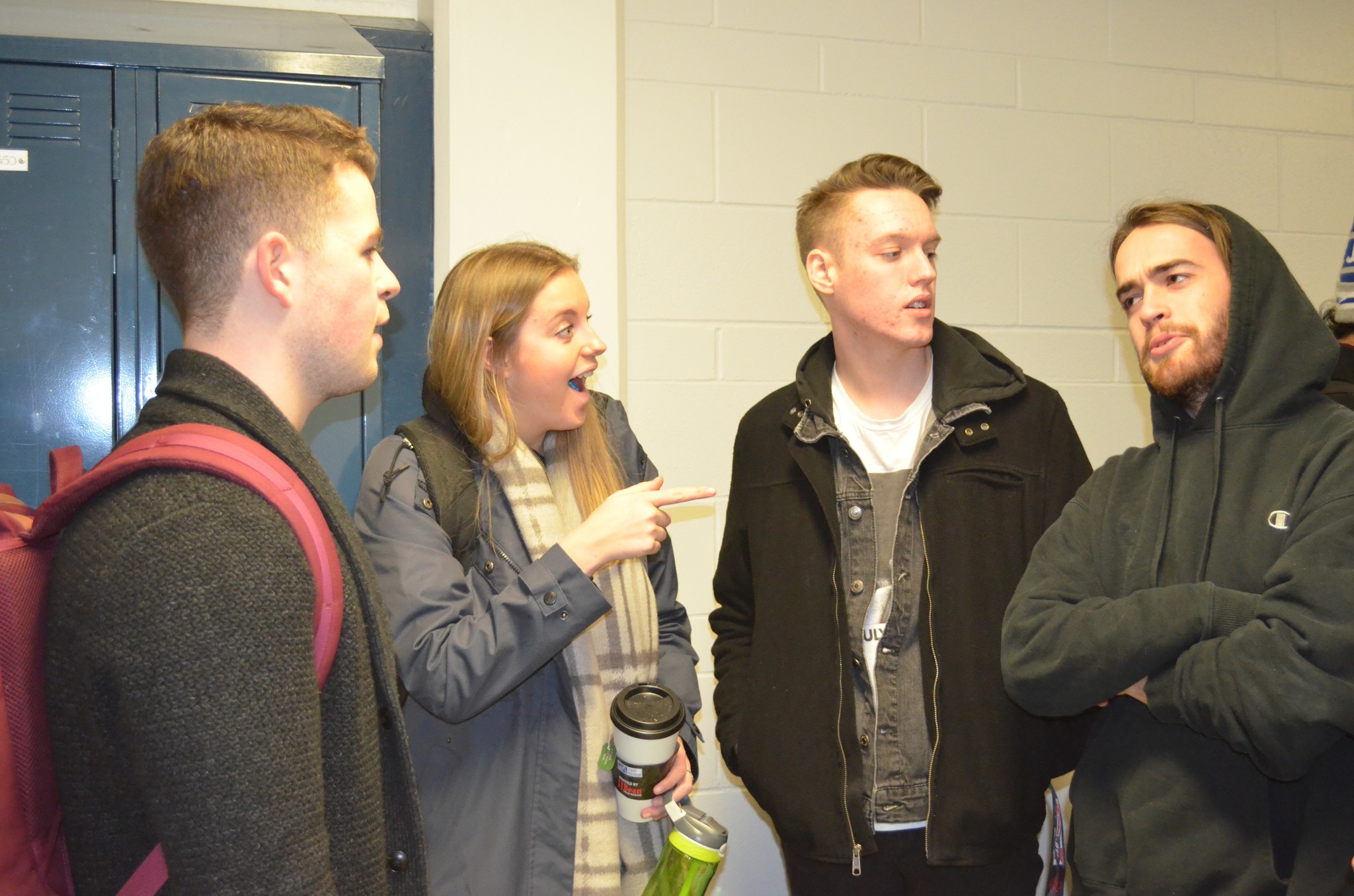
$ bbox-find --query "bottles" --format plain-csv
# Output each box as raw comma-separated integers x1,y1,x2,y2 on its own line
641,788,729,896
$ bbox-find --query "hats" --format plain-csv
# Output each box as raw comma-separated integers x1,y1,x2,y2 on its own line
1334,222,1354,324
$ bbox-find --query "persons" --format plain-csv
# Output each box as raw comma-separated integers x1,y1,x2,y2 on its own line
355,243,716,896
708,155,1093,895
1001,201,1354,896
43,104,433,896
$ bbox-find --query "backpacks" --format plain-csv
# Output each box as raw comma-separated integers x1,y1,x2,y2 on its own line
0,422,345,896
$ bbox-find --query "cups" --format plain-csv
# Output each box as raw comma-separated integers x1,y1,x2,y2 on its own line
609,681,687,822
